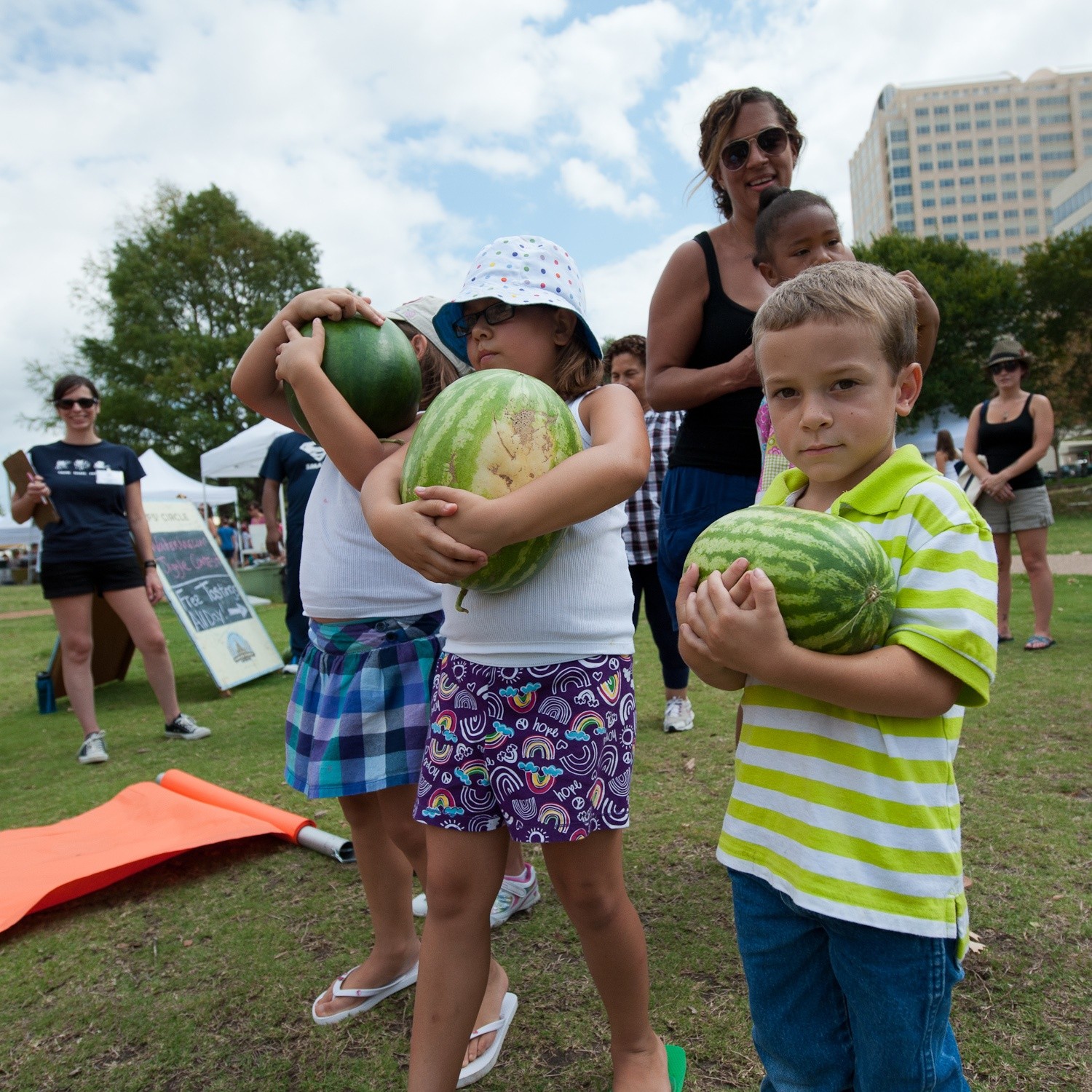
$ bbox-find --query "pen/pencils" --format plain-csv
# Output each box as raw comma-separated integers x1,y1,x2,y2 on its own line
26,472,49,505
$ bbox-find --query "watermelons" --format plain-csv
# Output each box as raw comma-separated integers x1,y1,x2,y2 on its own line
683,506,895,656
282,312,422,446
402,369,582,613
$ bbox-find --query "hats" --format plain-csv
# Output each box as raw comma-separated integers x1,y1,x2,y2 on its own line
979,340,1035,371
431,235,604,370
379,295,477,379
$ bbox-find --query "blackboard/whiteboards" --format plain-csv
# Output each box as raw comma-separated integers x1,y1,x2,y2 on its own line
47,500,285,699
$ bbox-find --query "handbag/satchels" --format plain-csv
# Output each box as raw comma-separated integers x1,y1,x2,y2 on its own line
953,399,990,506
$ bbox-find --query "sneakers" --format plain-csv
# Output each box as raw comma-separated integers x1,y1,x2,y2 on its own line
163,712,211,740
663,695,695,734
411,891,427,918
76,729,109,764
490,863,540,930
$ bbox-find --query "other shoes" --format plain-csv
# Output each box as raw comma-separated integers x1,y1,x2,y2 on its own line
281,653,301,675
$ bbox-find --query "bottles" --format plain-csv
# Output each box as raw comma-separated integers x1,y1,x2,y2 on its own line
35,670,57,714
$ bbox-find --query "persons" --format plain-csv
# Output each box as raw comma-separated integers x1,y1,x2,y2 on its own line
962,337,1057,651
934,430,962,481
218,88,938,1092
9,375,212,764
676,262,1000,1092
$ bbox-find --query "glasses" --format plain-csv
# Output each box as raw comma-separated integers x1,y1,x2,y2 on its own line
55,398,98,410
990,360,1022,375
247,508,254,511
452,301,519,338
718,127,791,172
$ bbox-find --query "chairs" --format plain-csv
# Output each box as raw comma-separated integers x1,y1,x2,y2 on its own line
240,523,268,566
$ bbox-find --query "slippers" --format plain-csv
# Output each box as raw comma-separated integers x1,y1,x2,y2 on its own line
312,957,420,1025
456,992,519,1089
1024,635,1056,650
998,634,1015,644
664,1044,687,1092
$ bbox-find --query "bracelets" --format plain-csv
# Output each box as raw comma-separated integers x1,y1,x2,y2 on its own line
144,560,156,567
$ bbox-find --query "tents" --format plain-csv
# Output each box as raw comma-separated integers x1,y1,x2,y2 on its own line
0,417,292,582
